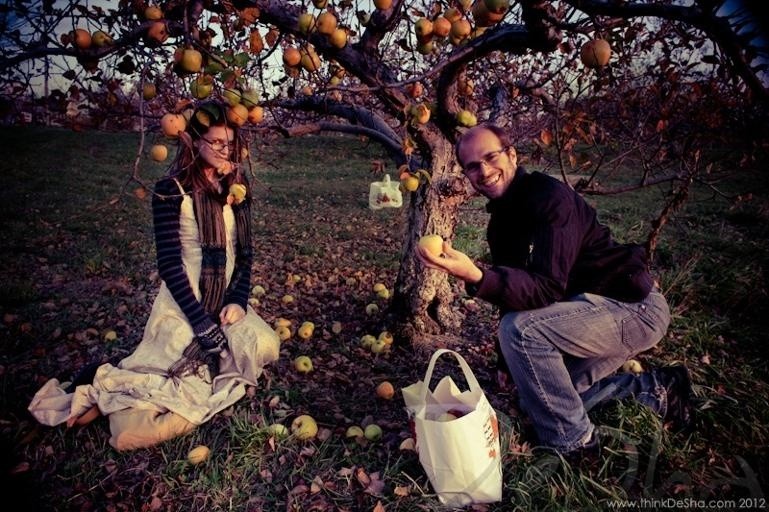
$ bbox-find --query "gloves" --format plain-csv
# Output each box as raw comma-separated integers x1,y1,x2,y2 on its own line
192,317,231,361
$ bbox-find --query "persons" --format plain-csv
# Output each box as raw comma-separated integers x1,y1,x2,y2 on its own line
415,123,694,467
64,103,70,104
26,100,282,452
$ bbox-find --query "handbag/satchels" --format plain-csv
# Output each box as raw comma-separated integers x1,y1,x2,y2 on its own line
400,348,504,509
368,173,403,210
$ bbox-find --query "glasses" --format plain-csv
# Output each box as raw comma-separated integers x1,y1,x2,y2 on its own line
202,135,236,151
459,146,510,176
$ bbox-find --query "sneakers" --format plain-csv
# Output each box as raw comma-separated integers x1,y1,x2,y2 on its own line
655,364,698,441
562,429,603,474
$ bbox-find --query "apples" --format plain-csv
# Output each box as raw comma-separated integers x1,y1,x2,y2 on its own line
68,0,642,468
